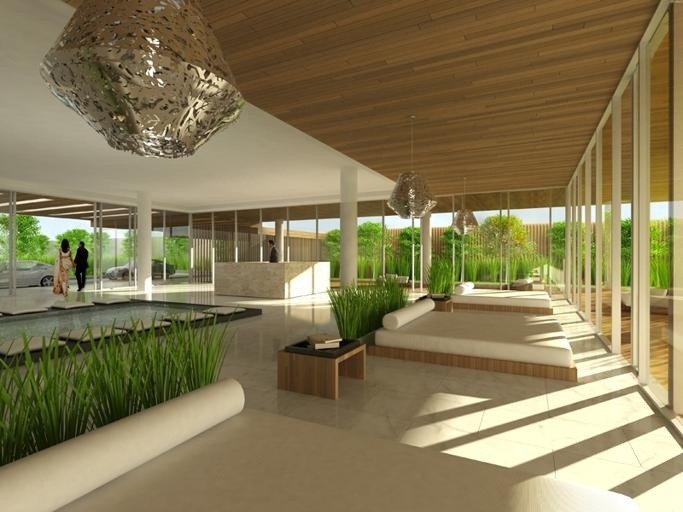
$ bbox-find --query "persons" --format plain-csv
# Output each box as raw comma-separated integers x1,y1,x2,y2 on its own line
74,241,89,291
268,239,279,263
56,239,75,297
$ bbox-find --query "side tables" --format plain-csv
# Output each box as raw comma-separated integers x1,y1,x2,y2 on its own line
277,332,369,403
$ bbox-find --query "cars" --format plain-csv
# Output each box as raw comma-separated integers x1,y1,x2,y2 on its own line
105,259,175,281
0,260,55,289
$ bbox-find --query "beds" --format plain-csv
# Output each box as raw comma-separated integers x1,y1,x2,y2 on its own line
0,374,643,509
372,280,574,384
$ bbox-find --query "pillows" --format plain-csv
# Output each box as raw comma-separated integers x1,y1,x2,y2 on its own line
1,375,246,510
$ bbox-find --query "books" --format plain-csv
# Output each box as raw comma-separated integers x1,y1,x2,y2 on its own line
306,332,343,350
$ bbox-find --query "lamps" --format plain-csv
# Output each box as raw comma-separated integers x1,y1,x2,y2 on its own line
450,177,478,237
38,0,246,158
386,112,436,220
